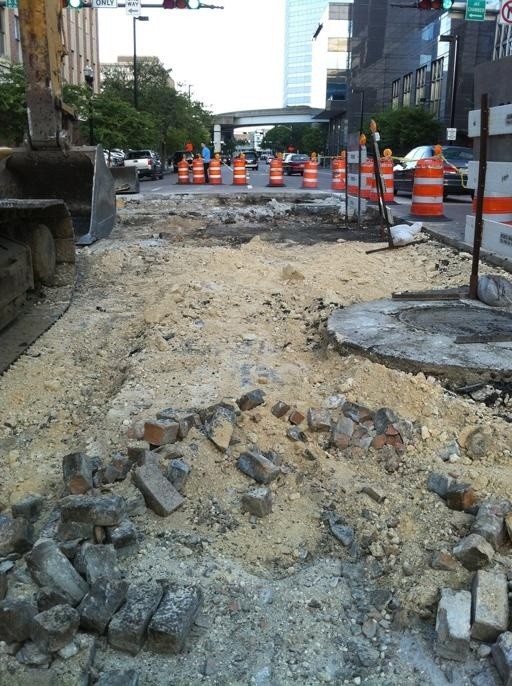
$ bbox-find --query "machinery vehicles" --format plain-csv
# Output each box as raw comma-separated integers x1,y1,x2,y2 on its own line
0,1,139,375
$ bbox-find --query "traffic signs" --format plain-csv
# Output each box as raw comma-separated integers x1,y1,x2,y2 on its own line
464,0,487,21
5,0,17,7
91,0,117,7
125,0,141,15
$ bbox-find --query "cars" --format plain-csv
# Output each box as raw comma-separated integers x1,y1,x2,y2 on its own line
103,147,164,180
393,143,479,200
173,150,195,173
220,153,231,165
239,150,259,170
261,152,311,175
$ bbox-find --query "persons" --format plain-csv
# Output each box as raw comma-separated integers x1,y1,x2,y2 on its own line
200,142,210,185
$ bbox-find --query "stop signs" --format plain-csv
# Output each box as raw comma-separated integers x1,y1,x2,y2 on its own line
287,145,295,151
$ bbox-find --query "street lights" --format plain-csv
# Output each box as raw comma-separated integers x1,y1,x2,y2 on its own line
83,64,94,145
132,15,148,108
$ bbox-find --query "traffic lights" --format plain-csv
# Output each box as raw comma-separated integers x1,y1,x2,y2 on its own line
163,0,201,9
69,0,82,8
417,0,455,10
